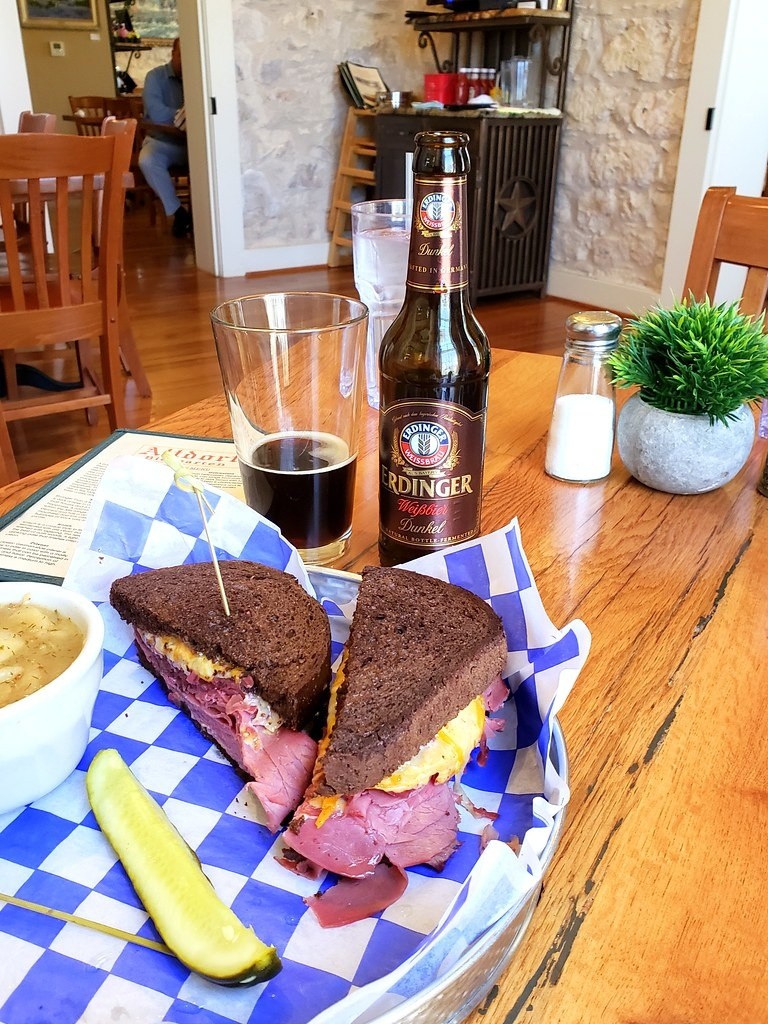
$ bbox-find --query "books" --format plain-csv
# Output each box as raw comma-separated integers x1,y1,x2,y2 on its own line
338,59,391,109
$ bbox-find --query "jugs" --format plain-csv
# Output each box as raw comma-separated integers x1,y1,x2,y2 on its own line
497,56,539,108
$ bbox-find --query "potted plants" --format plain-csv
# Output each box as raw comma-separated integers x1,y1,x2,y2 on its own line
603,285,767,495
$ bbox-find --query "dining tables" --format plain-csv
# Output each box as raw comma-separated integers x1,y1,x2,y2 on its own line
1,317,766,1024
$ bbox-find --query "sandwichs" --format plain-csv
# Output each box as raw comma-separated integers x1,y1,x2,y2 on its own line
285,566,506,881
111,561,332,826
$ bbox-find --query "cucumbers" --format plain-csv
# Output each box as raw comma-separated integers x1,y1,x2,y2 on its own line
89,746,284,987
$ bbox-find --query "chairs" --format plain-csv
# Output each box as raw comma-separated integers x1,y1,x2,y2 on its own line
0,87,192,484
680,185,767,336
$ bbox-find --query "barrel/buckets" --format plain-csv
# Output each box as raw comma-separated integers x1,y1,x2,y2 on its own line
423,73,456,105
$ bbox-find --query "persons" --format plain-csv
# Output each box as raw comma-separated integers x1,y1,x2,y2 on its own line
137,37,198,242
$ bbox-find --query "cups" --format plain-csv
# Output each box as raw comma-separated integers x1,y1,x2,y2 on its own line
352,199,413,411
209,292,370,563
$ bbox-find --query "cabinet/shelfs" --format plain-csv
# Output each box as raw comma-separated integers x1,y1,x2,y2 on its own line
375,105,565,305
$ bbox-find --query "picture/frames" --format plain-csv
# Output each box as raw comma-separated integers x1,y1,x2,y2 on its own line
16,0,101,32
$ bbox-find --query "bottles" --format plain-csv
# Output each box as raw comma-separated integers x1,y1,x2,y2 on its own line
457,68,496,105
545,311,622,484
381,129,494,566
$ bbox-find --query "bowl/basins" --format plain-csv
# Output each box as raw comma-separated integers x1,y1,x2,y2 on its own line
376,91,413,109
0,583,103,815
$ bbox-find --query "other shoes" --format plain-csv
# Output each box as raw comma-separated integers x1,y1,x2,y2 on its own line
176,216,192,231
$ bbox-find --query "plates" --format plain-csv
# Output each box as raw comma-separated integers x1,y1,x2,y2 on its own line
305,565,570,1024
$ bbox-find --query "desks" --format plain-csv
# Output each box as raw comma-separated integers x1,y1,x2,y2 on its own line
0,172,137,195
60,112,118,127
137,120,187,135
